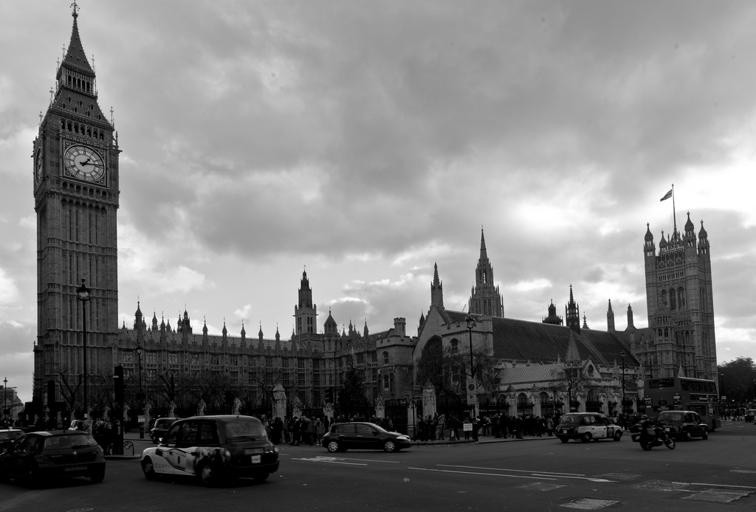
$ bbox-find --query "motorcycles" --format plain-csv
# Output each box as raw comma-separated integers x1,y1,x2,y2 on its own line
639,427,676,451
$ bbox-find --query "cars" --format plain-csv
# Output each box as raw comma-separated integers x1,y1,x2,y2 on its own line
319,421,413,453
554,411,626,443
647,410,709,441
135,414,281,489
0,427,107,490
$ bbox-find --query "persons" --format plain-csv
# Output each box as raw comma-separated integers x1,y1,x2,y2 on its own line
260,412,393,446
713,396,756,423
411,411,440,441
608,409,672,447
446,409,562,440
0,416,133,456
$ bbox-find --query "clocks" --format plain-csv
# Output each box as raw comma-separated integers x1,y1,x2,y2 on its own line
62,143,106,184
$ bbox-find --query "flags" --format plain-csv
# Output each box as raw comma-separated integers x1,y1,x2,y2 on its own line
660,189,673,201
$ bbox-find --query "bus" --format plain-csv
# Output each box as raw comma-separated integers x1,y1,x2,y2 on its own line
643,376,722,433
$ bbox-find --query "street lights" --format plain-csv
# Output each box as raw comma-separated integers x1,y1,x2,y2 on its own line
465,310,476,379
136,338,146,417
76,277,91,416
